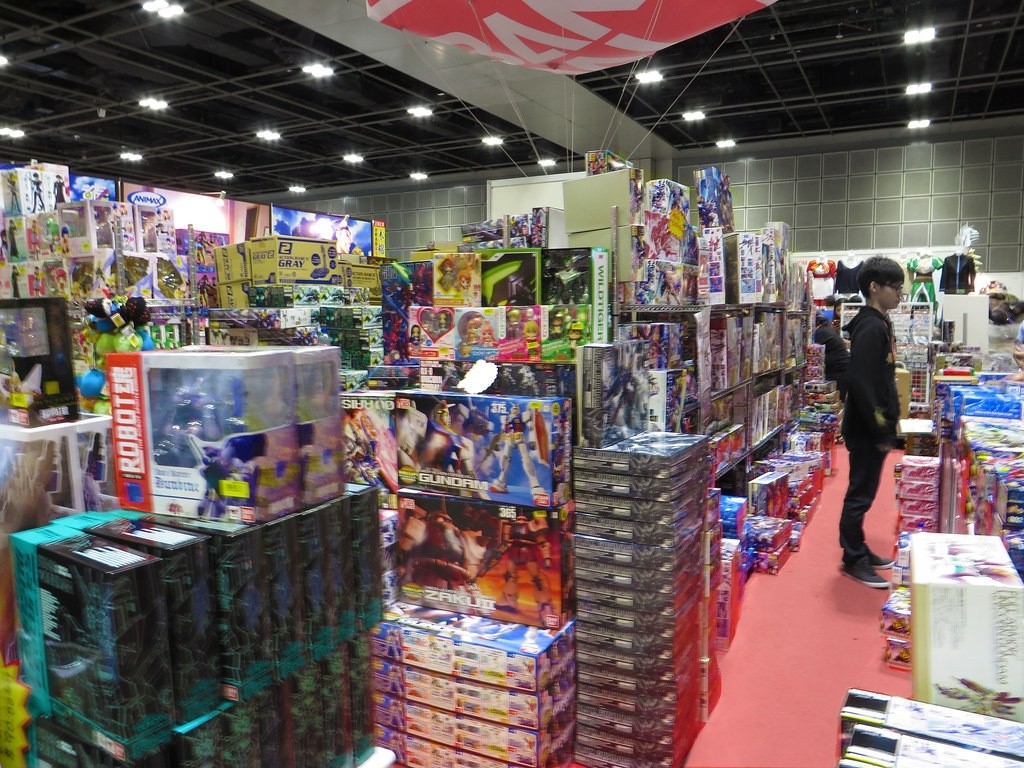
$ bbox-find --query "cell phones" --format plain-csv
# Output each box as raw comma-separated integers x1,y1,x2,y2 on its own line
833,320,840,325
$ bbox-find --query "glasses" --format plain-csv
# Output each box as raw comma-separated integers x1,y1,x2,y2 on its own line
876,282,903,292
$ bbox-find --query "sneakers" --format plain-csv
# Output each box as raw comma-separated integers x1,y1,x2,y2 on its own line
842,564,891,588
869,551,895,569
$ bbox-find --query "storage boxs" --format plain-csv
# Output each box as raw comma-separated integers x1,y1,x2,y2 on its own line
2,148,1023,768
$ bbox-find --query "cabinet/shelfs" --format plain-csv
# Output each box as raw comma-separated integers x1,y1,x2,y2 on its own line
748,302,789,482
786,305,812,426
611,300,753,502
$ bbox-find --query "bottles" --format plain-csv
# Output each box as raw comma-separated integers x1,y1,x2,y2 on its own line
915,521,926,535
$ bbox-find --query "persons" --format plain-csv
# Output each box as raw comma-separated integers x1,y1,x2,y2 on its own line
816,294,862,413
1003,319,1024,381
908,249,977,308
838,254,908,591
